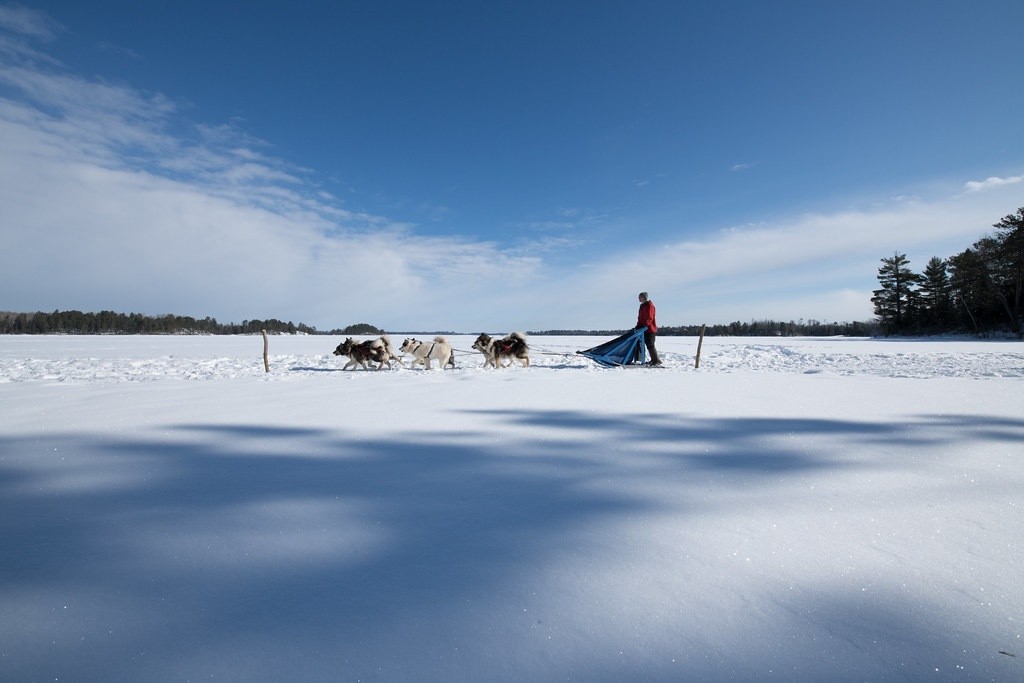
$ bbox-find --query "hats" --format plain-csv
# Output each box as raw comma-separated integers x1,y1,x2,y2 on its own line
641,292,648,300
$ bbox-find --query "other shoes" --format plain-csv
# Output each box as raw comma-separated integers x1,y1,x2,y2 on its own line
650,360,662,366
645,360,652,365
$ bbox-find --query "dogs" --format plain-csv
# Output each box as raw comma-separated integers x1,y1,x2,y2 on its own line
333,337,404,370
472,333,529,369
399,335,456,370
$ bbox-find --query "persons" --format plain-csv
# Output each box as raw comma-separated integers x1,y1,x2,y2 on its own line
636,291,663,366
